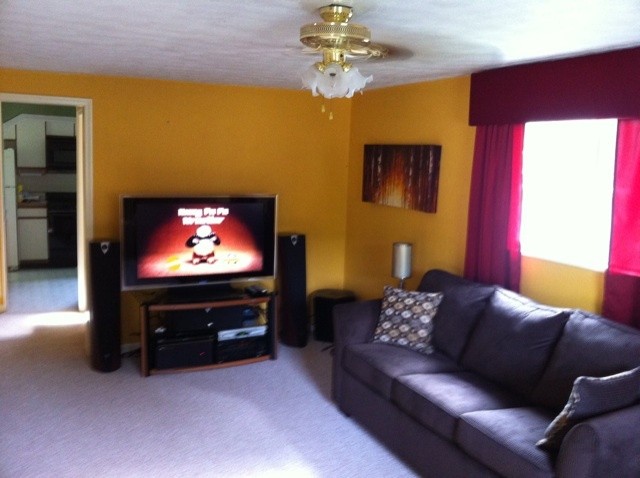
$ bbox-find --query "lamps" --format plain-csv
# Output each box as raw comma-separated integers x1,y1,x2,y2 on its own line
391,242,411,288
298,4,390,122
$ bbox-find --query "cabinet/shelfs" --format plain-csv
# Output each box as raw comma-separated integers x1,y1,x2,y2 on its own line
138,288,280,378
17,200,50,268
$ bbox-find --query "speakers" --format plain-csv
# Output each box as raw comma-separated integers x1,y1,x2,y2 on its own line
87,237,123,374
276,231,311,349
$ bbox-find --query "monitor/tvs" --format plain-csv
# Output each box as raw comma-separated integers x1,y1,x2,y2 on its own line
118,192,279,304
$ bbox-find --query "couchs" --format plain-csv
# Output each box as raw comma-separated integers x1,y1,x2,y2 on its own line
331,267,638,477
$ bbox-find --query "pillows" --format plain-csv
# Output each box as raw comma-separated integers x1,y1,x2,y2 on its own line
370,285,442,354
536,368,638,447
